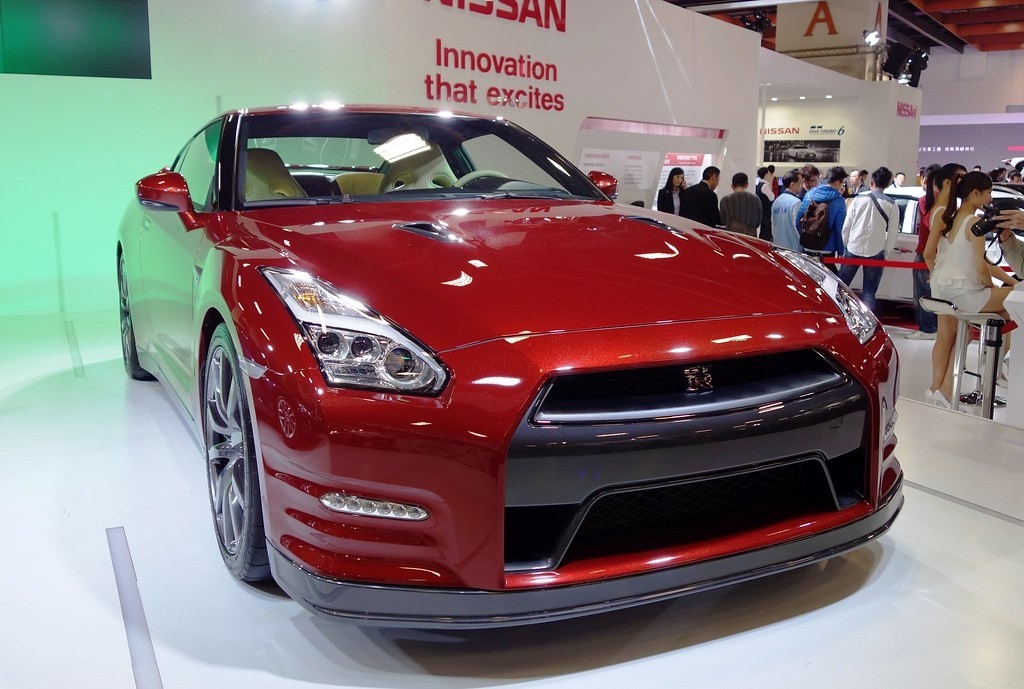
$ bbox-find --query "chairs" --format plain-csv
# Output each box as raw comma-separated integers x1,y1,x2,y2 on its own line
245,147,308,202
379,139,460,195
331,172,384,195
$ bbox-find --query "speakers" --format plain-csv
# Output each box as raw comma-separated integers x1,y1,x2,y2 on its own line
882,42,914,78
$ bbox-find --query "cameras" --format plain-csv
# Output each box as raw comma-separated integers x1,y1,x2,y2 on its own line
971,204,1006,237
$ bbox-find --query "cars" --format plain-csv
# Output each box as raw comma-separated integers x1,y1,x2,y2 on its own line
114,98,909,631
787,145,816,160
832,183,1023,309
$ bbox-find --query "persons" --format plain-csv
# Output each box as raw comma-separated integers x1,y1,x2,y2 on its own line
659,160,1024,414
838,168,899,309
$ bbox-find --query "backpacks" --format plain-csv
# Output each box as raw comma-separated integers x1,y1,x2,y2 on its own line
799,197,833,250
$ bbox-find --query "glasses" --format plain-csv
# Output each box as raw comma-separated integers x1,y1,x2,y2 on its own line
842,182,847,187
805,178,820,183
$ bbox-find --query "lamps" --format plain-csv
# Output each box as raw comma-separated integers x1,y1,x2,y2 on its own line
861,27,882,46
897,70,912,84
916,47,928,59
373,128,434,164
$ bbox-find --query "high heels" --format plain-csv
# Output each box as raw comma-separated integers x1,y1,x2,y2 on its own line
926,388,941,407
932,388,966,413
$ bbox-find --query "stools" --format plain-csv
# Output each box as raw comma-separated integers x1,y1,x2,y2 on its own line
919,293,1007,420
